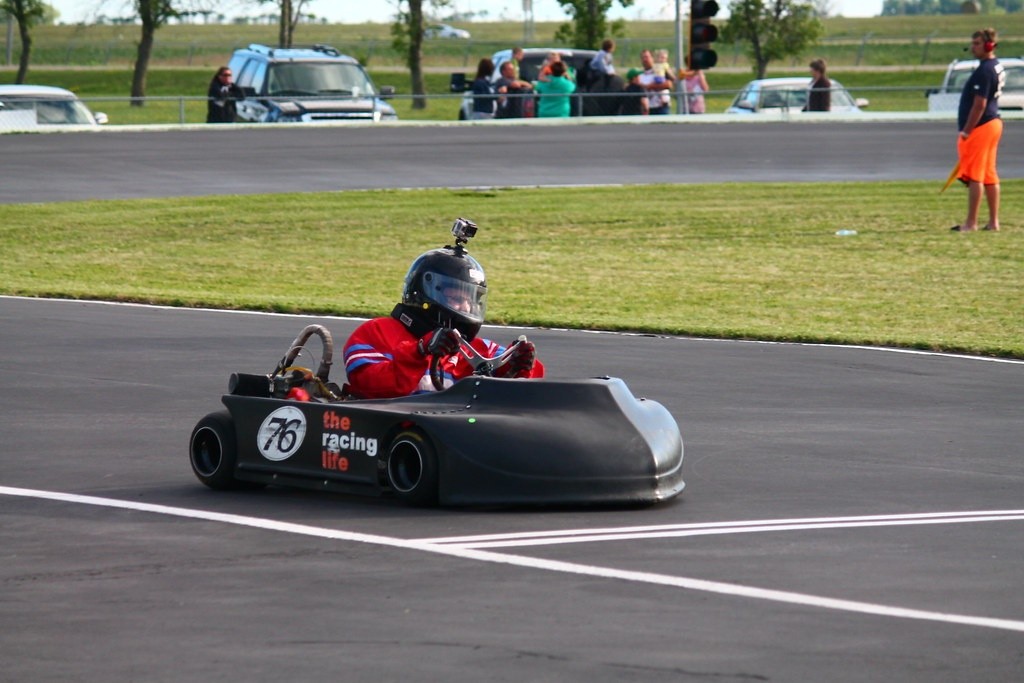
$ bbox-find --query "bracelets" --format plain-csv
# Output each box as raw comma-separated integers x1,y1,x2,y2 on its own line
960,130,968,137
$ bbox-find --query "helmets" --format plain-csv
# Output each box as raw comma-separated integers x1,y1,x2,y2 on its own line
402,248,487,343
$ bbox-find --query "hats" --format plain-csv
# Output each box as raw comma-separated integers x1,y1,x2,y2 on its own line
627,68,644,79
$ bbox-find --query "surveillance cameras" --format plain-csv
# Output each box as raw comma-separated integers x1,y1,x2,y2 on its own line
452,218,477,238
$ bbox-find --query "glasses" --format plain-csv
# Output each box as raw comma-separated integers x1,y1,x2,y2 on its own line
221,73,232,77
445,295,471,304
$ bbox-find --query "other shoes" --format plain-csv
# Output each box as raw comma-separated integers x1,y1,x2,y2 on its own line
981,224,988,231
950,225,960,231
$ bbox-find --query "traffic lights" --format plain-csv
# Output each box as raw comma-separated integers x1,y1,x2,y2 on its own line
687,0,720,72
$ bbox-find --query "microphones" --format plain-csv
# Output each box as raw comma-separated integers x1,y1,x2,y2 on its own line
963,47,970,51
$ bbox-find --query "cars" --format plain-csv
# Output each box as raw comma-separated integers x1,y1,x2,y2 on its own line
1,83,113,128
723,77,870,117
422,23,471,41
448,47,622,119
926,56,1024,115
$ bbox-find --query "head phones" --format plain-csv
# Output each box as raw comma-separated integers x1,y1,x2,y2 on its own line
981,31,995,51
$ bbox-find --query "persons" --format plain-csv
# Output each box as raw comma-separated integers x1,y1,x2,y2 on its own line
950,27,1007,233
495,62,533,119
590,39,616,75
206,68,244,123
533,52,576,118
638,48,676,115
685,55,710,113
342,248,545,399
472,58,504,119
510,47,524,79
801,58,831,111
620,67,649,115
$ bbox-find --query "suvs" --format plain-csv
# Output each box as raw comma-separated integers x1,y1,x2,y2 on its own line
220,44,401,124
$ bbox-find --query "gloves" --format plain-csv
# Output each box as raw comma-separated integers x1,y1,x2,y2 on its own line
509,340,535,371
417,328,459,358
